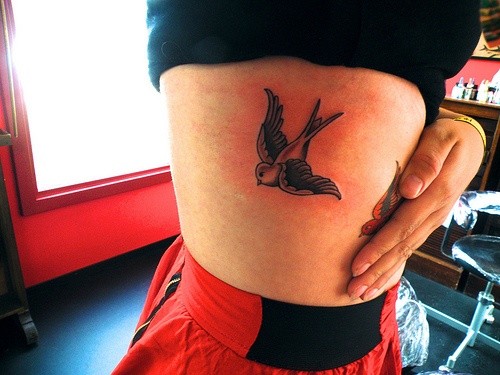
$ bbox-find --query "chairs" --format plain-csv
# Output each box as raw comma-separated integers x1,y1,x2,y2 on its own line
411,190,500,375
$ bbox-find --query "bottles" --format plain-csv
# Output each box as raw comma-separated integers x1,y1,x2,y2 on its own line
464,77,475,100
457,77,465,100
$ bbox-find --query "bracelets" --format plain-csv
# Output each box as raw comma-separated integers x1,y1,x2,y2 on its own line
453,115,487,152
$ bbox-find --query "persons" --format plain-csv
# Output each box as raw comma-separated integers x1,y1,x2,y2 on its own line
111,1,489,375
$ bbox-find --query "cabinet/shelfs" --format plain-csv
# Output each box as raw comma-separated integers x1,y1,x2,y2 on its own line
1,130,40,349
405,95,500,314
